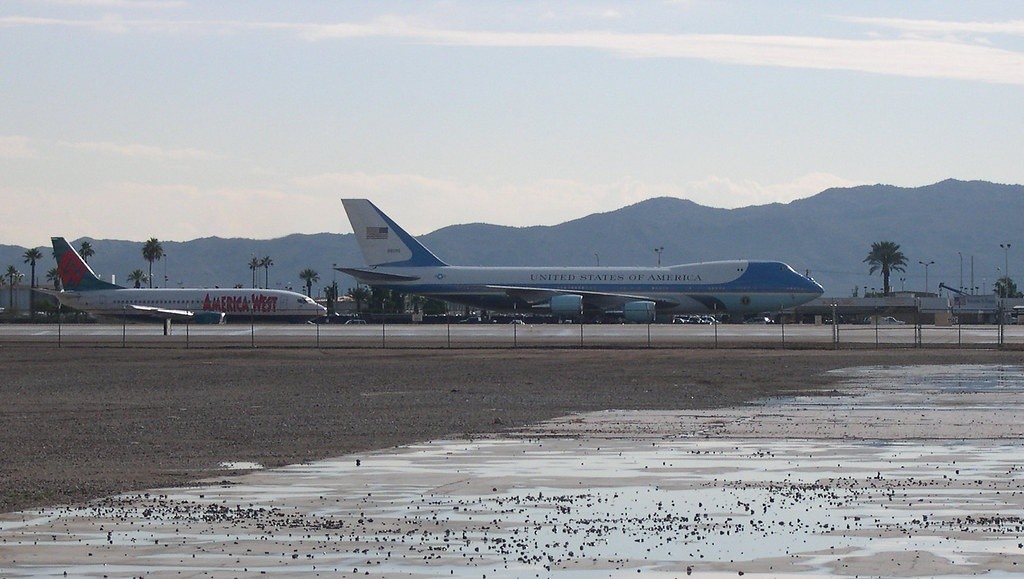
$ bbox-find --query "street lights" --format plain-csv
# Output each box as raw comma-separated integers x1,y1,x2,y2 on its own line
829,303,837,344
958,252,962,296
595,252,601,267
900,278,905,291
162,253,168,288
1000,244,1011,309
919,261,935,297
654,246,664,266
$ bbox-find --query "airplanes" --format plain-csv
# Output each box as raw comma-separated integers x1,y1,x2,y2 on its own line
335,197,823,324
29,236,329,325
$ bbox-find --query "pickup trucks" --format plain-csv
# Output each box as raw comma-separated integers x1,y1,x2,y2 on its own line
879,316,906,325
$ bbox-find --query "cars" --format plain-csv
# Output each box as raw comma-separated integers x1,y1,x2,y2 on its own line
459,317,483,323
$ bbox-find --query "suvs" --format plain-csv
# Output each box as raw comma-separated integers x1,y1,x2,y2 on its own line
345,319,366,325
